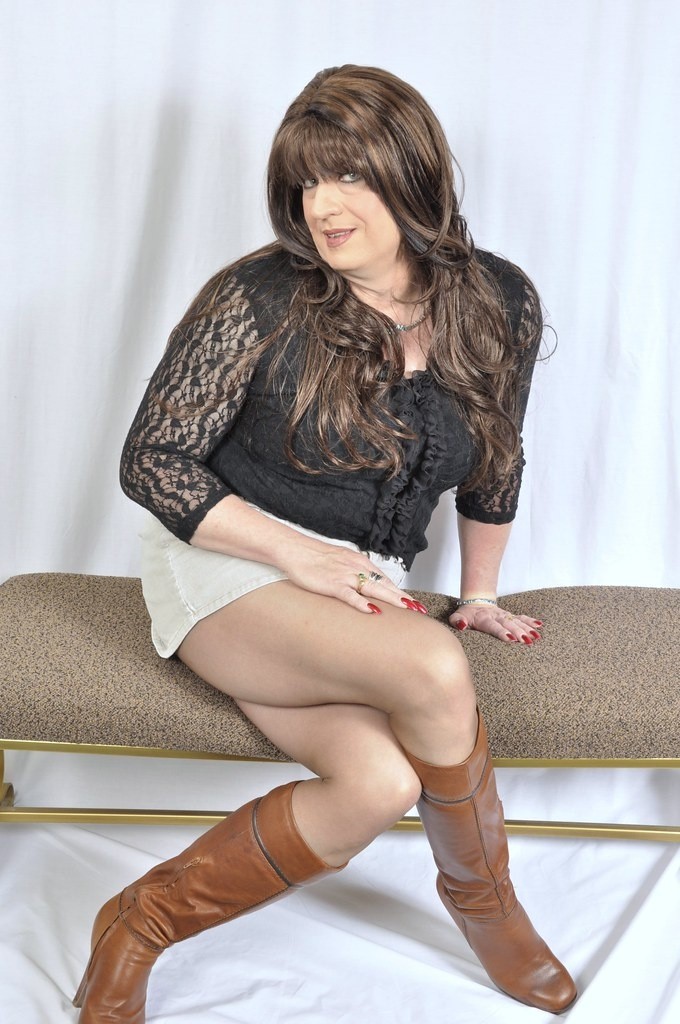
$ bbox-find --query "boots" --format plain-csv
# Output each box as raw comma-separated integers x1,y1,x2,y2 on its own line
72,780,350,1024
397,704,577,1015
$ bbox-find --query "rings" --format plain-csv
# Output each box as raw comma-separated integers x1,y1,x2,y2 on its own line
356,573,368,594
506,615,514,621
368,571,383,583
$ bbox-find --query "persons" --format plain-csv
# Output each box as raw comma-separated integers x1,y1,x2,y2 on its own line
71,65,577,1024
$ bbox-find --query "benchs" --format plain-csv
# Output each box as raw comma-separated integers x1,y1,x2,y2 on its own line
0,572,680,846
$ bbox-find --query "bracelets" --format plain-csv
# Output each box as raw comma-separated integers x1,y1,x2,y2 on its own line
456,598,497,606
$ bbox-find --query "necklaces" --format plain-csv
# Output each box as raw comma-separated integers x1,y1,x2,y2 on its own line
394,306,433,331
